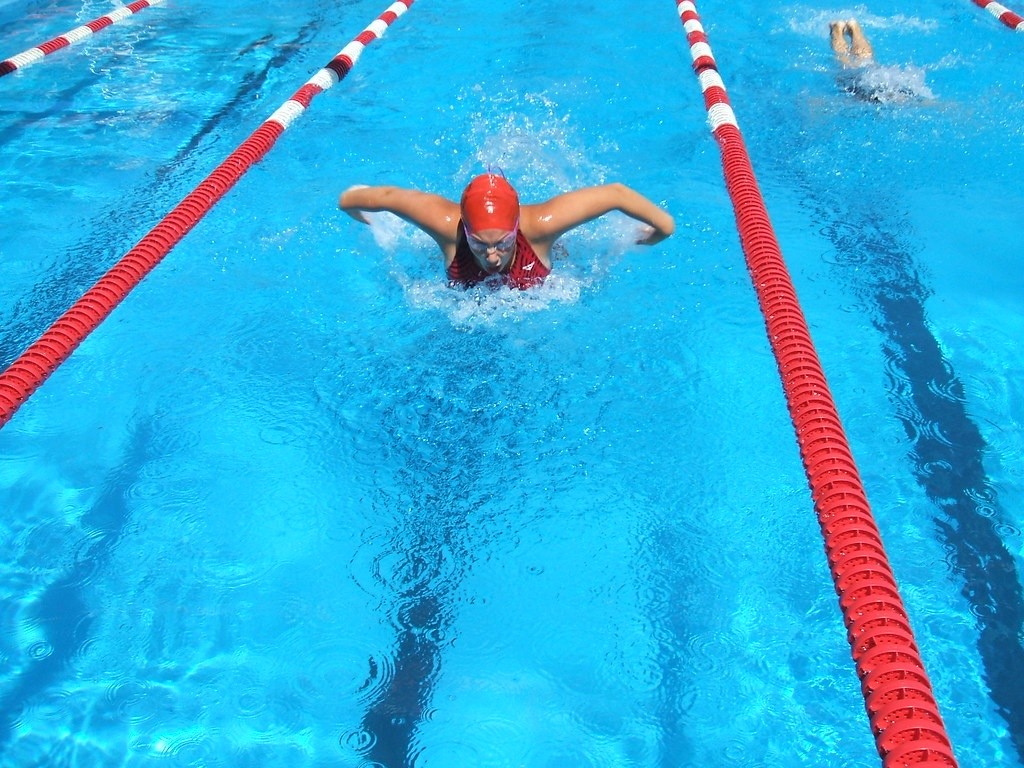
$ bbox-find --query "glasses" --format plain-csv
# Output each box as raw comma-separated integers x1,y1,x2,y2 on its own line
462,217,519,250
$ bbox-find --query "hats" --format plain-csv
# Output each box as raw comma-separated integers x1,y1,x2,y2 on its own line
460,173,520,233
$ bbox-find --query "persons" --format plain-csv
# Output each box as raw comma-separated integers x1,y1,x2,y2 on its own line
828,19,871,51
339,173,676,290
